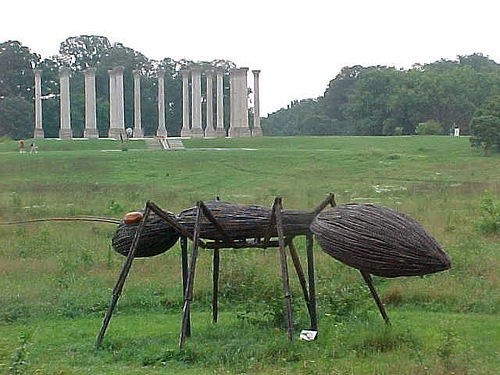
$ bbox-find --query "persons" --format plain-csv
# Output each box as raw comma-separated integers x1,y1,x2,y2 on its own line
19,141,25,154
29,141,37,155
